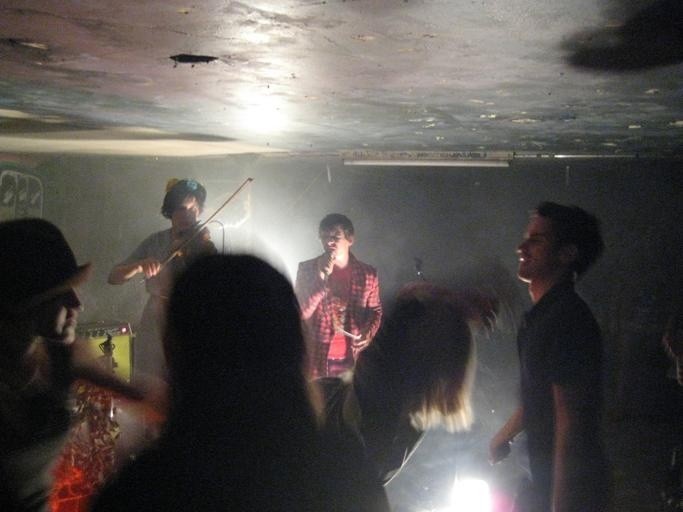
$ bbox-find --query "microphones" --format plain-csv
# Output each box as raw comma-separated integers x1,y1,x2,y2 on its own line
322,252,336,284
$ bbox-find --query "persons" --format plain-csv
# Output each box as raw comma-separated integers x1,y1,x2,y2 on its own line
0,216,142,511
82,251,390,510
296,214,386,387
484,202,603,511
102,179,218,380
302,284,500,484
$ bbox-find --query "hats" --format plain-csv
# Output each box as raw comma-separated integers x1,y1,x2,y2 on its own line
0,219,91,322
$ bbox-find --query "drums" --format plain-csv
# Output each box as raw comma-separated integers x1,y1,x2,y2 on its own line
75,320,134,393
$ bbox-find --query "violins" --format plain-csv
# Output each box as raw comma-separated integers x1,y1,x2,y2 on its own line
175,226,212,268
